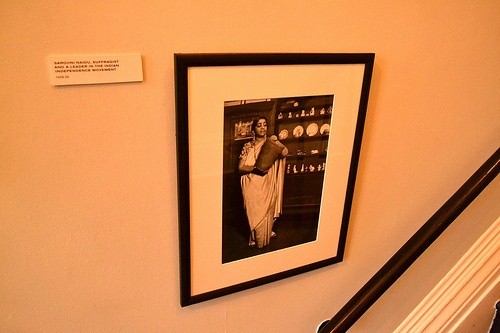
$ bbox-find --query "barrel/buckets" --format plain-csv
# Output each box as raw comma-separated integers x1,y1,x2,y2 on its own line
255,137,284,172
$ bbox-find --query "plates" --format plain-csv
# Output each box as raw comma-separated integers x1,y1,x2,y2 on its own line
293,125,304,138
306,123,318,136
320,123,329,134
278,129,288,140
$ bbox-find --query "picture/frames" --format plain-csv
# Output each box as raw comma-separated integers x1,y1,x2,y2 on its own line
174,51,375,308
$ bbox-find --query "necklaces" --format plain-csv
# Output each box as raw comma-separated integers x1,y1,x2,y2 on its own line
253,138,265,161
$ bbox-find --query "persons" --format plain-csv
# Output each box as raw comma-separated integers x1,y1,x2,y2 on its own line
237,115,288,249
